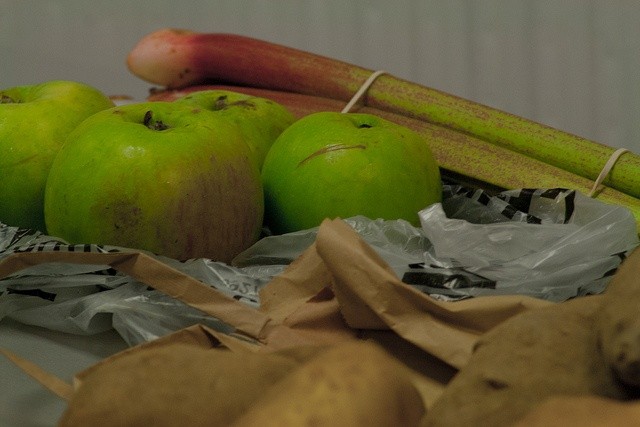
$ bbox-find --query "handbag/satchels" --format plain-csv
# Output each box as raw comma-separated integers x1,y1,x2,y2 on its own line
0,217,640,427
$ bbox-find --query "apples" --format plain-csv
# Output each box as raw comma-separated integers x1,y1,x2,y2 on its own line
177,90,296,157
43,103,264,265
0,81,116,237
262,112,443,229
